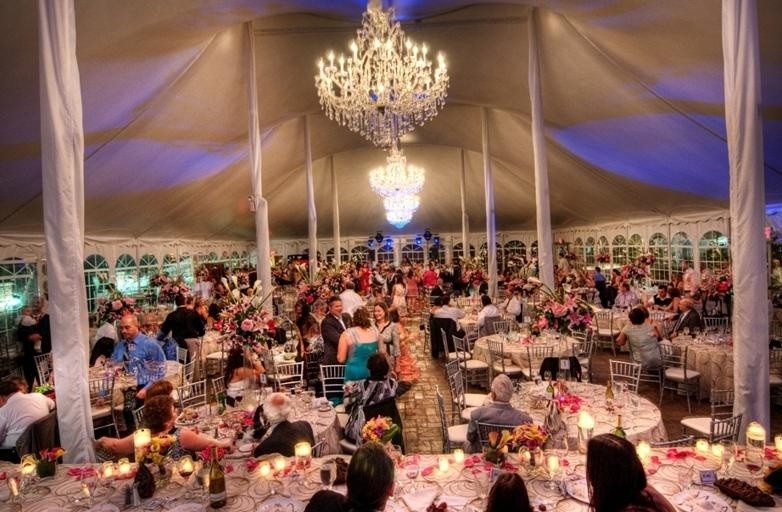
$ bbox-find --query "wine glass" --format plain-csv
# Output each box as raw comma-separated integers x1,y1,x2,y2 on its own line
684,327,690,336
693,327,700,337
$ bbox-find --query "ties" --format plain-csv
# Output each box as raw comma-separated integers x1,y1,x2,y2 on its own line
121,343,134,371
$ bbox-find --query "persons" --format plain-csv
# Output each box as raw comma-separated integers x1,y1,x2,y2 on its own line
0,253,781,512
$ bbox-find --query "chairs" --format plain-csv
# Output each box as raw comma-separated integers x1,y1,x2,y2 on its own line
0,258,782,510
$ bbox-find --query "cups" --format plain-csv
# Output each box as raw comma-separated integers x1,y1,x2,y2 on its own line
0,414,782,512
280,384,332,416
510,371,639,418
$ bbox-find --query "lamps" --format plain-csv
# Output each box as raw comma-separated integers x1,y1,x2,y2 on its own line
365,233,393,247
382,197,421,230
413,231,440,244
365,150,427,201
310,1,452,149
